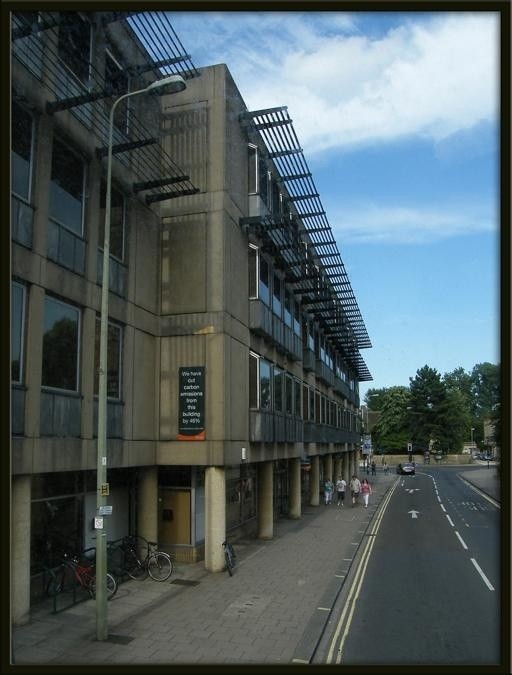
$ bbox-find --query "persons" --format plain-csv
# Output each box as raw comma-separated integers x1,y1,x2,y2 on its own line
349,474,361,505
371,461,377,476
360,478,372,508
382,461,390,476
322,478,335,505
335,475,347,507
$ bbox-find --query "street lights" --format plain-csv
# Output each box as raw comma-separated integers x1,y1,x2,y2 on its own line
90,72,193,646
361,392,381,465
470,427,476,446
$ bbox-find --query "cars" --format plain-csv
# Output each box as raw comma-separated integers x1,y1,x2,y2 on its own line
396,462,417,475
471,451,494,460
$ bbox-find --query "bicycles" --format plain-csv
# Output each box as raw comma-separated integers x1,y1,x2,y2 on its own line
221,533,239,577
41,532,174,601
